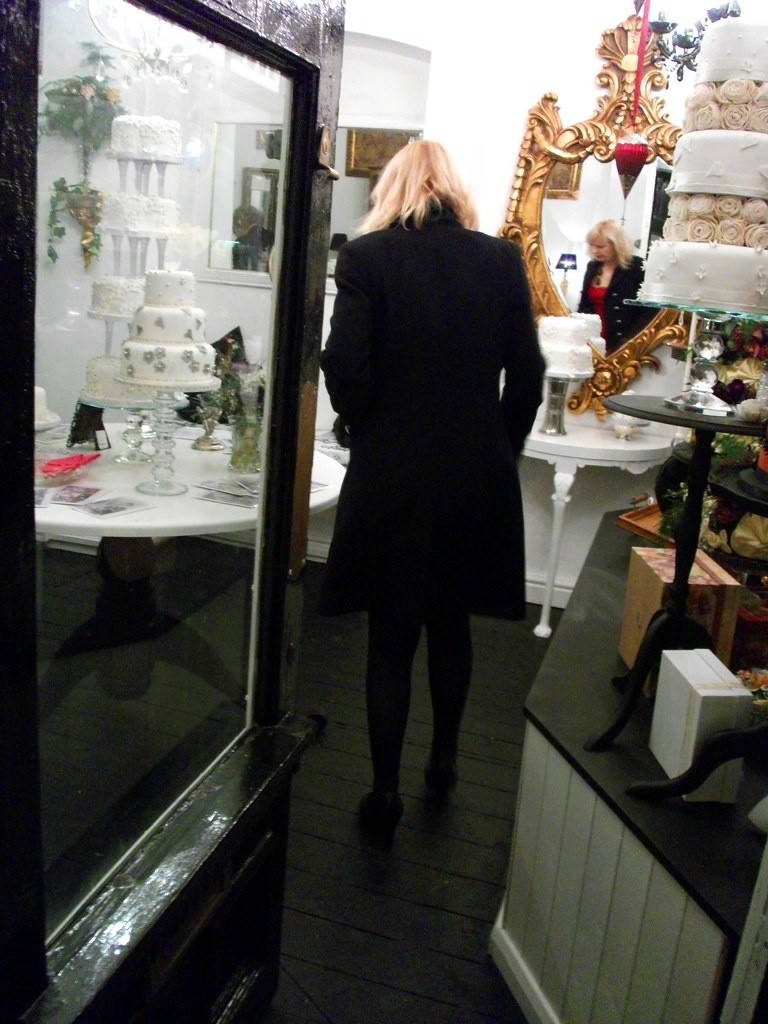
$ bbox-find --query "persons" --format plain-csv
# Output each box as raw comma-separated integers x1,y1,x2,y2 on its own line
321,135,551,828
576,219,650,352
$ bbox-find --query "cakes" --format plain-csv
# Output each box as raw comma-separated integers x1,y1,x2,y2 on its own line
637,17,768,311
77,111,220,404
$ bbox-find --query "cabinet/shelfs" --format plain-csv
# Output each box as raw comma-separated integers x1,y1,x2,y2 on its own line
483,507,768,1024
518,422,686,612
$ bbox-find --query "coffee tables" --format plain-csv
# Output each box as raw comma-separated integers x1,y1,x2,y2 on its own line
29,420,347,726
582,395,766,793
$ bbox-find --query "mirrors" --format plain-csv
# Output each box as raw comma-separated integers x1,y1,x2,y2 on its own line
500,8,675,421
195,112,435,298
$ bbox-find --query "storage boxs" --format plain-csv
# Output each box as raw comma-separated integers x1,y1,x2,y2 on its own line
647,648,755,805
616,547,742,702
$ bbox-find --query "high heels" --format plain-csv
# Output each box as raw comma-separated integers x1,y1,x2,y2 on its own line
358,792,405,853
423,765,457,798
